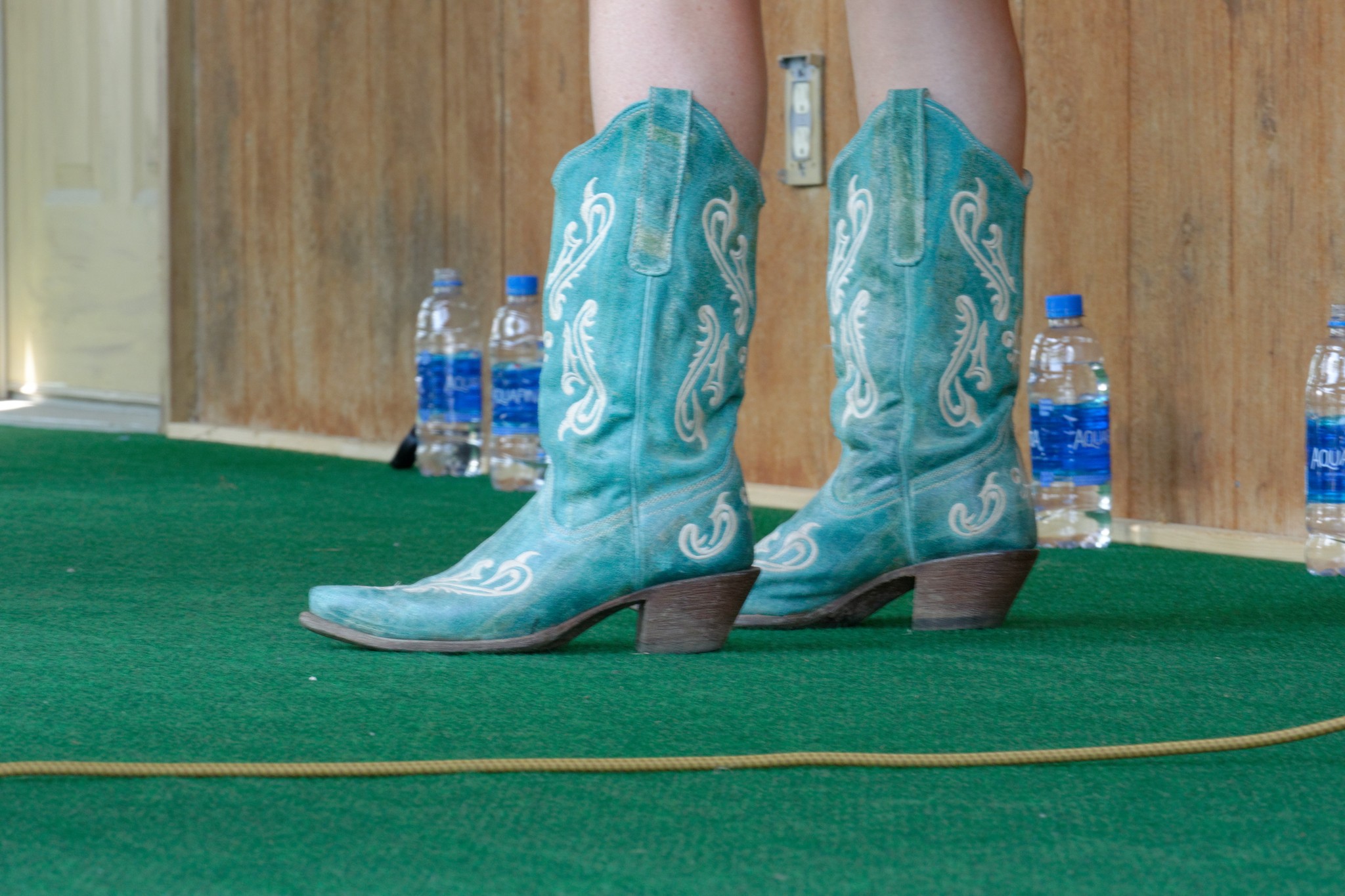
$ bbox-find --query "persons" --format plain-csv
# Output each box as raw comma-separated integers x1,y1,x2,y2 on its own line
298,2,1043,646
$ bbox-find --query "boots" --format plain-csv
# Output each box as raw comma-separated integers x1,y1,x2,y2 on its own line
731,87,1039,631
299,87,761,655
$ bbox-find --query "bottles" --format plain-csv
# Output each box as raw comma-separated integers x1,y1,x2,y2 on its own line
1304,303,1345,581
1028,293,1110,550
412,269,482,478
487,277,547,492
